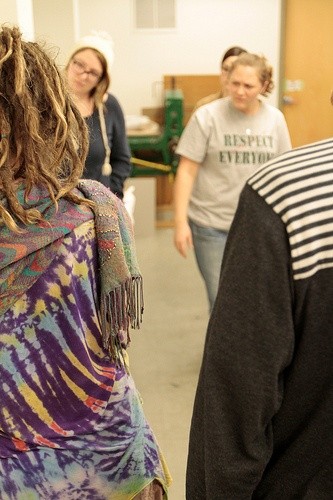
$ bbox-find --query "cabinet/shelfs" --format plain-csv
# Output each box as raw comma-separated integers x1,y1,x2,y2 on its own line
157,74,220,227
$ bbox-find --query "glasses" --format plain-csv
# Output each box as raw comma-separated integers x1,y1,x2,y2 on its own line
73,57,103,82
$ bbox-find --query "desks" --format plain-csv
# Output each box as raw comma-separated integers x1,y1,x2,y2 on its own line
130,118,162,178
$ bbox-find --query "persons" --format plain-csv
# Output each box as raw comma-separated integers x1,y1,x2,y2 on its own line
61,47,249,203
186,137,333,500
0,25,172,500
171,53,293,311
